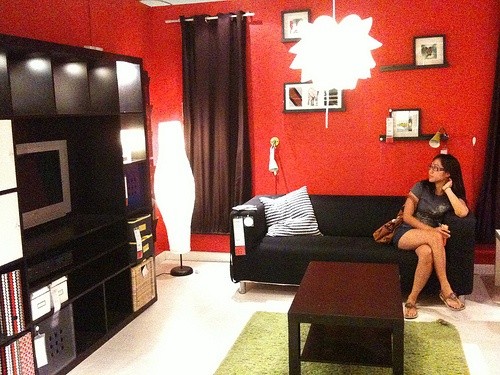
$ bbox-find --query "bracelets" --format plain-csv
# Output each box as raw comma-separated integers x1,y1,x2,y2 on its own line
444,187,450,191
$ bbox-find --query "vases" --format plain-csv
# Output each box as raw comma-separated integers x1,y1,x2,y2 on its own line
154,121,196,276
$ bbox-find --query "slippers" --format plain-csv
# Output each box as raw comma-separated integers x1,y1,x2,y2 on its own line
404,302,420,319
438,290,465,311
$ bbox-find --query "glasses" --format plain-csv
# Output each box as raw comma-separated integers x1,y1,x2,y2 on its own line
427,165,446,172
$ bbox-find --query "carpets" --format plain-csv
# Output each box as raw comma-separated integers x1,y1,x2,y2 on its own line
214,311,471,375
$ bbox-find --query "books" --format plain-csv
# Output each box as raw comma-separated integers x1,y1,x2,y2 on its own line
0,269,26,375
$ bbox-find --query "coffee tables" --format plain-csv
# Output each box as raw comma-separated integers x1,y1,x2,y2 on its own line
287,261,404,375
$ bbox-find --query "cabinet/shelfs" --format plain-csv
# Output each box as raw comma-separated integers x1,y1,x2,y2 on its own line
0,33,159,375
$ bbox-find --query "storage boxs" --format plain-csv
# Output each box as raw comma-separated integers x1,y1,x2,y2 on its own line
49,276,68,308
30,286,51,321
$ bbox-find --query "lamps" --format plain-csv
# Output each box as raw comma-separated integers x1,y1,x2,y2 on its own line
288,0,383,91
428,127,448,148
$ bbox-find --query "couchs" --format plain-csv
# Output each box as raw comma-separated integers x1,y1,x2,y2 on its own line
229,195,477,304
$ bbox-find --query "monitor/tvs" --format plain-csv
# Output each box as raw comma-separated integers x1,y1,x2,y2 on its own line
16,139,72,235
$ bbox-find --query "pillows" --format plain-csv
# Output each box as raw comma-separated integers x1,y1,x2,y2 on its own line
258,185,324,238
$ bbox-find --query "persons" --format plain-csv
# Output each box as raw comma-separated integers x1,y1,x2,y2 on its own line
392,154,469,319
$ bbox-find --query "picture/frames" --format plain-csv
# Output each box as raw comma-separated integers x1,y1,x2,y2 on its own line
413,34,446,67
281,8,311,42
390,108,421,138
284,81,346,113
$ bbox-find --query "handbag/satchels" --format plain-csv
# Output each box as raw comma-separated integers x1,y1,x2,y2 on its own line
373,195,418,245
